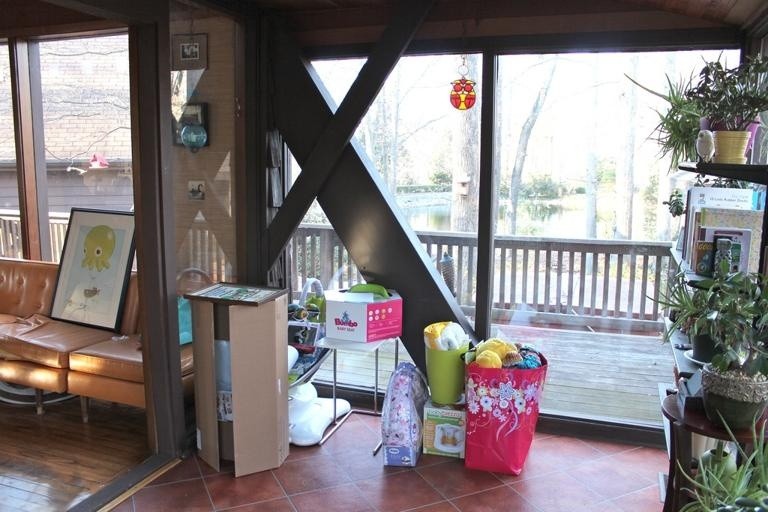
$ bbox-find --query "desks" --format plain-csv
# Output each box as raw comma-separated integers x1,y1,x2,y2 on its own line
313,337,400,456
657,393,767,512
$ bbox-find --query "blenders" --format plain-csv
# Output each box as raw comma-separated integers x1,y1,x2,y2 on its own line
438,425,465,448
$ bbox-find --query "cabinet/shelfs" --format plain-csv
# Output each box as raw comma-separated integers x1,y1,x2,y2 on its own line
661,159,767,444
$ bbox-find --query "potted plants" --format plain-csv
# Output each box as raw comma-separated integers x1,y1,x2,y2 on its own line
643,257,766,512
623,37,768,163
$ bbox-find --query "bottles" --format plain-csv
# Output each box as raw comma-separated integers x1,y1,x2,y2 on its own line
714,237,733,280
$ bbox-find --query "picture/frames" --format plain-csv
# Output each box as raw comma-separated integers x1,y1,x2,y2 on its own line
185,281,290,305
50,206,137,334
171,103,207,146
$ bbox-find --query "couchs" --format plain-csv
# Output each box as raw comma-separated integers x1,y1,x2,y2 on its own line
0,260,195,423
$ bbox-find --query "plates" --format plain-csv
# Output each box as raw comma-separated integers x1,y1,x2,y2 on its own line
683,348,708,366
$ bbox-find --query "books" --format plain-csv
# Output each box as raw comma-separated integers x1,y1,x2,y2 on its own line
683,188,765,289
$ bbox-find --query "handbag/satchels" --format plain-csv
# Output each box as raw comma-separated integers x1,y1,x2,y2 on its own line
380,360,429,468
460,343,549,477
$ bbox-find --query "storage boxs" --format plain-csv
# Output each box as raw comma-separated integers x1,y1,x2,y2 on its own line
187,296,290,475
423,398,467,460
319,287,405,341
